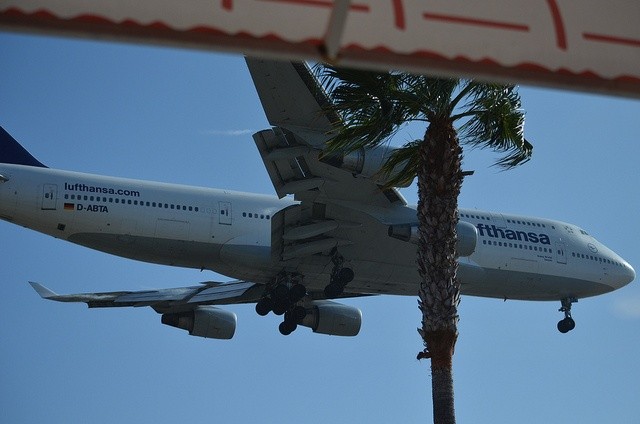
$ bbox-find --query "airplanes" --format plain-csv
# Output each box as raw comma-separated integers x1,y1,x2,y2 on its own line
0,56,635,339
